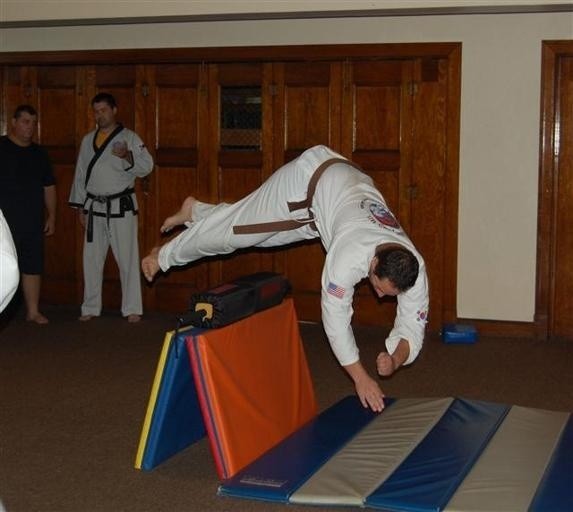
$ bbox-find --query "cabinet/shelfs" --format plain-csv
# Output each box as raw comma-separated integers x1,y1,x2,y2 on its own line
87,60,147,308
146,64,206,312
206,60,275,284
1,63,31,307
276,61,340,331
340,56,447,332
31,62,87,305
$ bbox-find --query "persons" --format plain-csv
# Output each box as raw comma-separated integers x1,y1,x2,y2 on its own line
138,144,432,413
1,104,60,325
66,92,154,325
1,207,23,316
